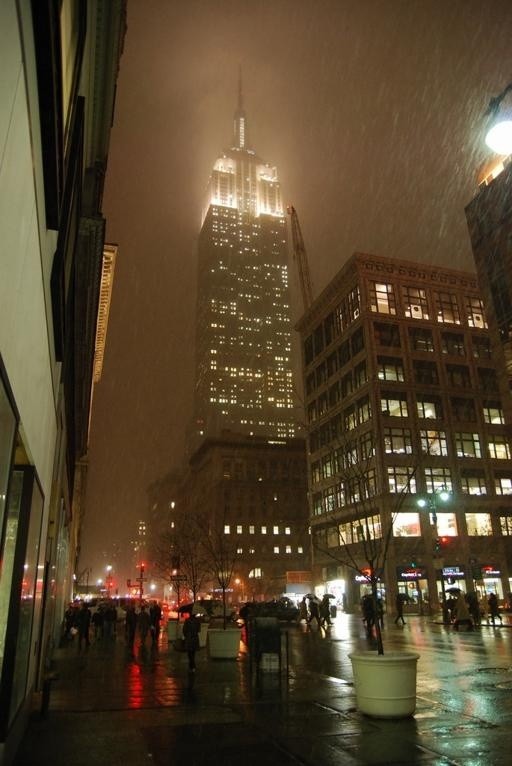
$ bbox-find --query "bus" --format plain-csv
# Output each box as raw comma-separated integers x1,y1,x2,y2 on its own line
265,569,315,609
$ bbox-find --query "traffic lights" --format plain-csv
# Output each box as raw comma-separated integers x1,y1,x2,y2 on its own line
109,582,113,587
140,561,144,572
434,536,441,552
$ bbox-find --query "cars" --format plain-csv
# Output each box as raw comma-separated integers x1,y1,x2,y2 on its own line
235,596,304,623
80,595,239,623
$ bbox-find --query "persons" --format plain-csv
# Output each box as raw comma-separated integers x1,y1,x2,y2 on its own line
395,593,406,625
442,592,481,631
64,598,162,647
488,593,502,623
296,595,333,626
363,596,383,632
182,613,201,668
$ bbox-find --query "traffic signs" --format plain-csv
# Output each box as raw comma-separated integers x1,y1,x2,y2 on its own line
135,577,148,582
169,575,187,581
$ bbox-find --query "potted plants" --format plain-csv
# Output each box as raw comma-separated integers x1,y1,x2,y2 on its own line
279,370,455,718
148,493,250,660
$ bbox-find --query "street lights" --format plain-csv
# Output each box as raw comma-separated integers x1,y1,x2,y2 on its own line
106,563,113,598
417,482,454,624
